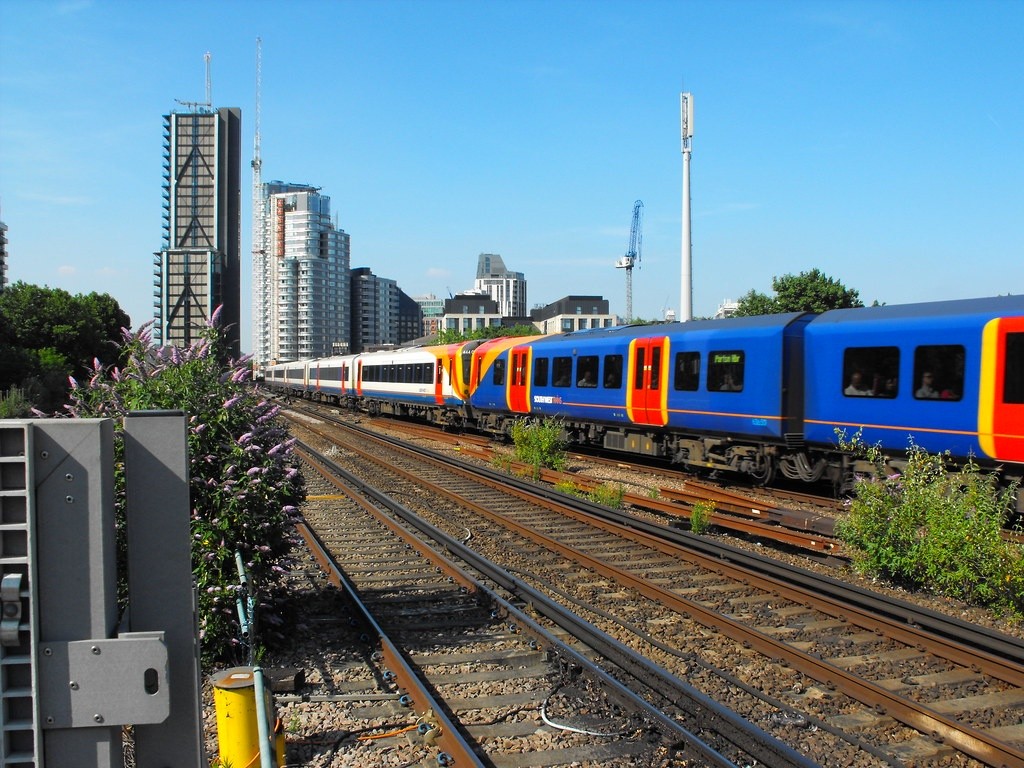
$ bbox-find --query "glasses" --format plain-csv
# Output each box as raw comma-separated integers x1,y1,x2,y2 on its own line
924,376,932,379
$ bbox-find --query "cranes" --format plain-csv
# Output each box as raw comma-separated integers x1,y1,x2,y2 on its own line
615,199,643,323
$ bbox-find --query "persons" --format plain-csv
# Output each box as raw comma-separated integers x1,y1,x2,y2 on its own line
556,372,943,398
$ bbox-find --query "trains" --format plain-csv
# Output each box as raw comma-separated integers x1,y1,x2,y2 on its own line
261,295,1024,532
